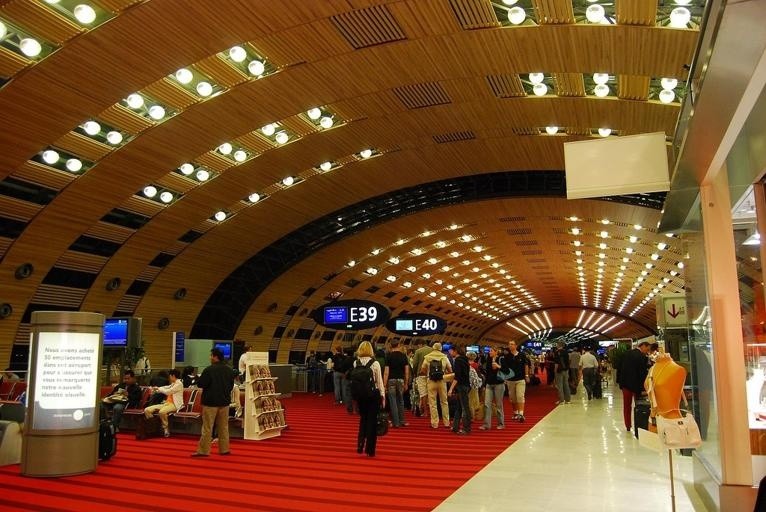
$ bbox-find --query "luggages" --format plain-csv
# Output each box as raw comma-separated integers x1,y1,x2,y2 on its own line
411,391,424,416
99,417,117,460
634,395,652,439
592,376,602,397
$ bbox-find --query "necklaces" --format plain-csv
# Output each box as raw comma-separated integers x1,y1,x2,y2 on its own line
648,360,672,413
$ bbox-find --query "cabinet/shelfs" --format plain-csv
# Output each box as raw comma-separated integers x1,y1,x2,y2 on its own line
244,352,287,440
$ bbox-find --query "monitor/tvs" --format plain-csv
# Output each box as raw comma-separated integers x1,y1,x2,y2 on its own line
437,340,542,356
213,340,233,359
323,306,349,325
103,316,130,350
394,319,414,333
131,317,143,350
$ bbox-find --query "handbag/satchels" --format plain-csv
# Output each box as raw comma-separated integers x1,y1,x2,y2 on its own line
134,413,176,439
497,368,515,381
656,412,702,448
376,416,389,436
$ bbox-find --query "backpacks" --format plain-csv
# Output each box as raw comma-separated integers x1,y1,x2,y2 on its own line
351,358,376,399
103,391,129,404
143,393,167,407
429,360,443,382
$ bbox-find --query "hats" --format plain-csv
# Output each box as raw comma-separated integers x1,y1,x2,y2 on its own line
390,339,398,344
433,342,442,349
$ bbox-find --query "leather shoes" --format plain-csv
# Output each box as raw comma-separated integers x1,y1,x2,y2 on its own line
357,442,375,457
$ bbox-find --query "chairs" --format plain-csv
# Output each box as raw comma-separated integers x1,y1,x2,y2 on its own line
0,380,245,438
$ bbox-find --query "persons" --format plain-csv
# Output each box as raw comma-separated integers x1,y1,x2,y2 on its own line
102,370,142,432
150,370,170,387
190,348,232,459
643,354,687,434
144,369,185,437
307,338,659,456
233,369,241,386
211,384,242,443
4,369,20,382
180,366,199,387
238,344,253,384
13,388,26,406
130,349,151,376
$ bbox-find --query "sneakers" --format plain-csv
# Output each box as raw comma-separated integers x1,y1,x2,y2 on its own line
478,425,490,429
555,400,572,405
191,453,209,457
497,425,504,429
217,451,231,455
457,428,472,434
519,415,525,422
511,414,519,420
